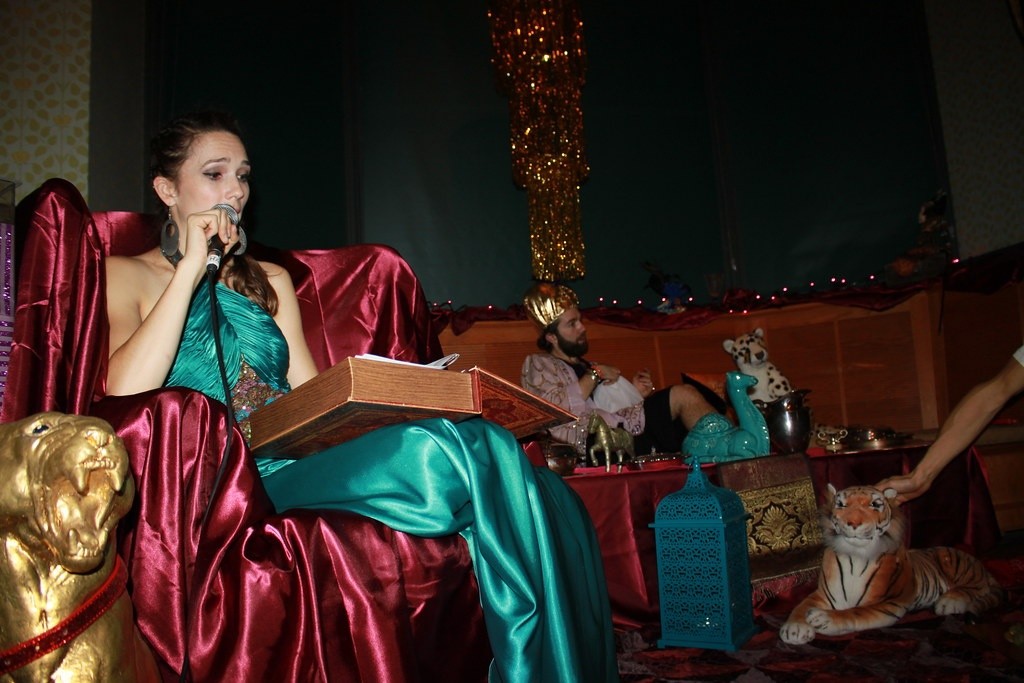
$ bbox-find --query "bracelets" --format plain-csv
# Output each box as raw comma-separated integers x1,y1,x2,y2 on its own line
586,364,604,385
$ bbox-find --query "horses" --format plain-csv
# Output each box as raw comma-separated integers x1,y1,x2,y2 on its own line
587,409,635,473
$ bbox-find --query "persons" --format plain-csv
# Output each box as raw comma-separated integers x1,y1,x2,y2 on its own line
105,103,617,683
878,344,1024,506
520,282,719,459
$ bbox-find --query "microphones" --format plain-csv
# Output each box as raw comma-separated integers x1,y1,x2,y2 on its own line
207,204,240,269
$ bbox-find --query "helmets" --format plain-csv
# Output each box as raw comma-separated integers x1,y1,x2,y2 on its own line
524,283,577,330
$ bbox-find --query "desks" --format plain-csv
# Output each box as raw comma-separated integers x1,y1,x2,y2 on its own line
545,439,966,627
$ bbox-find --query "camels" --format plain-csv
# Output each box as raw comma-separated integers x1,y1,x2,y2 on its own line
682,371,771,465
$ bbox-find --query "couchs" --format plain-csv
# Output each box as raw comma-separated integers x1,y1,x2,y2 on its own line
12,271,950,683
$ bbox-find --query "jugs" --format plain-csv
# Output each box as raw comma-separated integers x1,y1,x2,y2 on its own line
752,387,813,457
